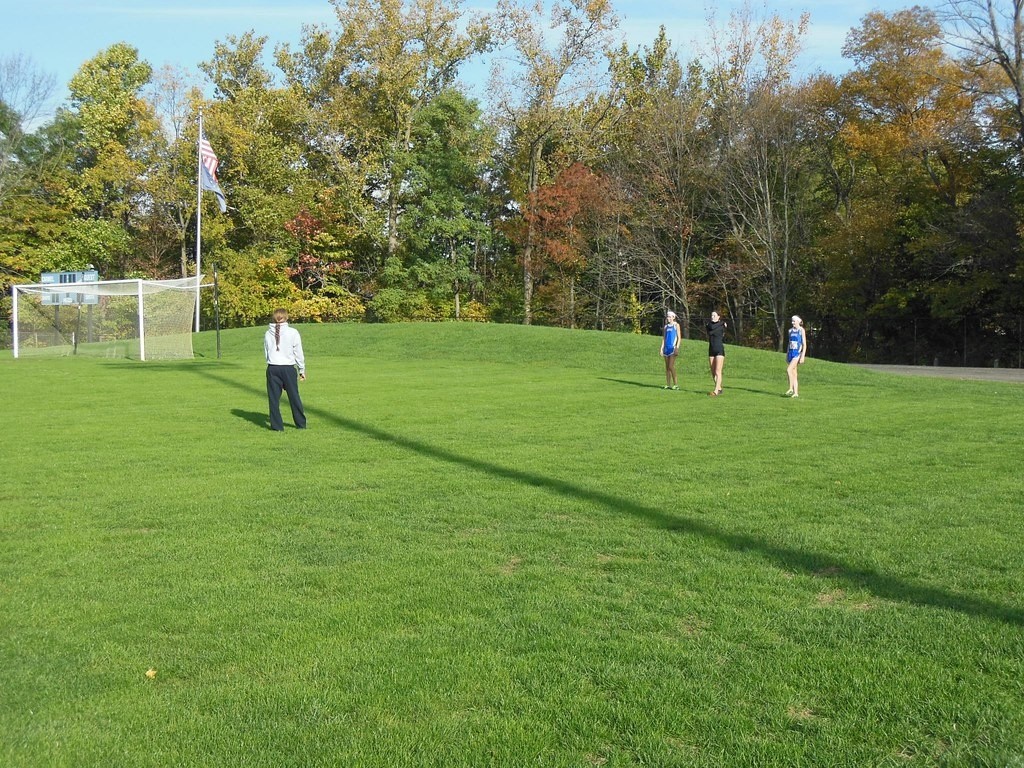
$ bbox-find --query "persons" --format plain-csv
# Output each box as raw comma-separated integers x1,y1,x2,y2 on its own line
264,308,306,431
659,312,681,390
786,315,807,397
706,310,728,396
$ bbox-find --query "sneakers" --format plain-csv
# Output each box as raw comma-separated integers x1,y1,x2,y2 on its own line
663,385,670,389
671,385,680,390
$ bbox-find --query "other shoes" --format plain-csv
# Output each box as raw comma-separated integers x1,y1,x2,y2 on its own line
785,388,794,395
707,391,718,396
790,394,799,398
718,389,722,394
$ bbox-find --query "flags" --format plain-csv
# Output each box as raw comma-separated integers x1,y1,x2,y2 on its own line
201,128,227,213
